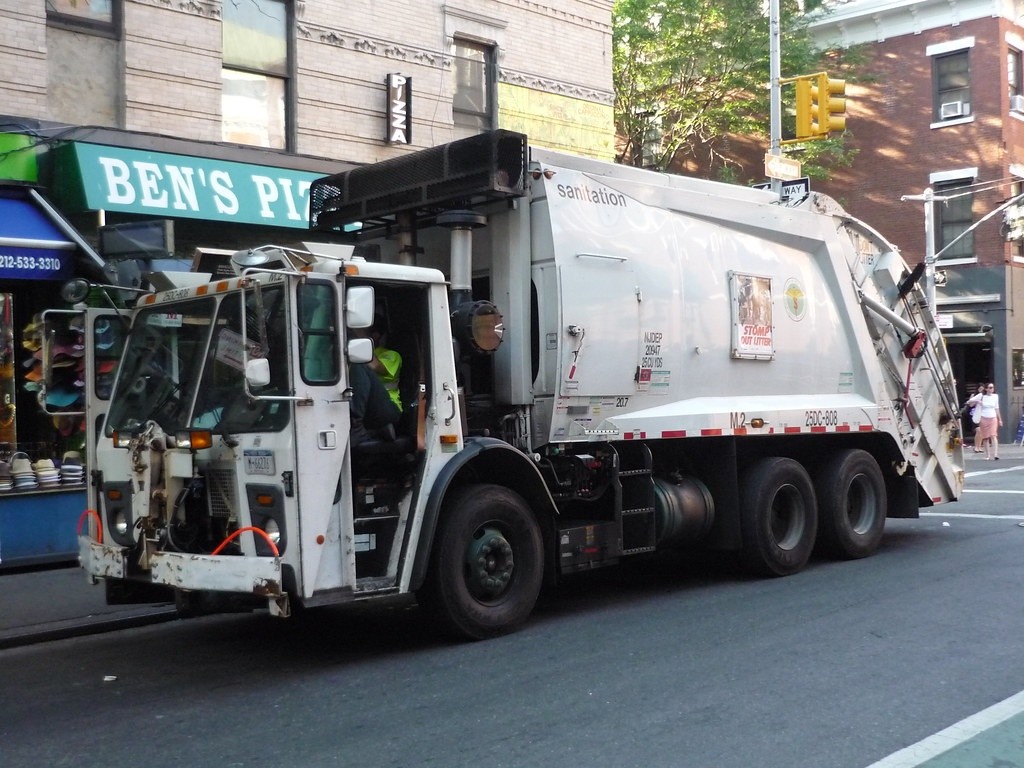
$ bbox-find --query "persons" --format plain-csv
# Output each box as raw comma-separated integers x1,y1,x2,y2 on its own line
214,363,283,409
967,383,1003,461
346,313,403,448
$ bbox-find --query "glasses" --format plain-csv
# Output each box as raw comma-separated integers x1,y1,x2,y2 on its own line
986,387,994,389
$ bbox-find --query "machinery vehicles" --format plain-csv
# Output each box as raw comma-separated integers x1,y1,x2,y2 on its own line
74,127,966,644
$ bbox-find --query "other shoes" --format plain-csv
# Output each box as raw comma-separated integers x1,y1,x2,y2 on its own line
350,423,371,448
378,423,396,441
987,457,990,460
994,457,999,460
974,446,984,453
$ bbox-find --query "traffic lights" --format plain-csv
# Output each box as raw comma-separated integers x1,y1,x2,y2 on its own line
826,72,847,138
808,80,820,137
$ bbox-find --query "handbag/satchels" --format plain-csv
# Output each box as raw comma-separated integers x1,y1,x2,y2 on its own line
972,394,983,425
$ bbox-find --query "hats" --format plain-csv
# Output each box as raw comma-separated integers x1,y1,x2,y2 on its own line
22,305,115,434
0,449,85,492
